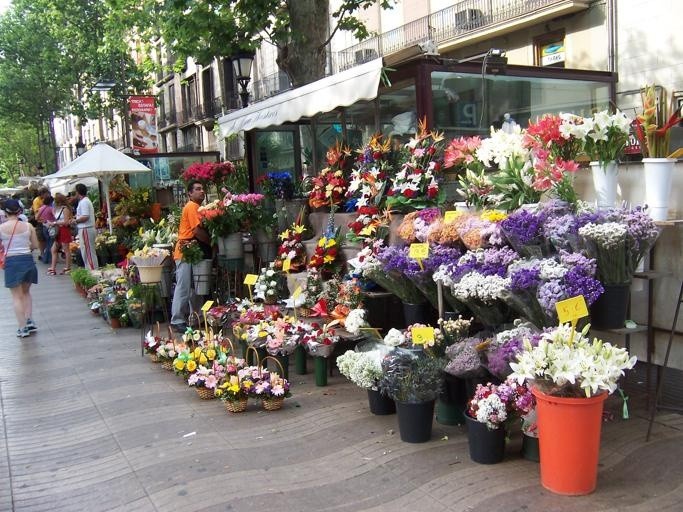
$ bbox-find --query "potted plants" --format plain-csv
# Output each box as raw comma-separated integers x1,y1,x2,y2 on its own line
84,277,96,294
70,267,87,292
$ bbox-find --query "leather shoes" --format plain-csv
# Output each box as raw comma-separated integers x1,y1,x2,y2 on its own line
193,319,211,328
172,323,194,332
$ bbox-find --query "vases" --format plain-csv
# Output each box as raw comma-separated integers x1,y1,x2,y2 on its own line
117,244,127,263
255,344,268,369
521,432,539,462
191,256,212,297
365,388,396,414
314,357,328,387
255,227,275,263
111,317,119,329
396,209,442,245
639,158,678,222
588,160,618,208
274,354,289,386
151,202,161,222
435,394,463,425
530,383,609,497
238,340,253,366
590,283,629,328
393,398,435,444
293,345,308,375
402,302,428,327
216,232,244,260
430,309,440,329
463,408,507,463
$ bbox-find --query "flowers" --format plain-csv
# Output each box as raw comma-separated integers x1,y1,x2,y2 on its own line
513,379,538,437
254,262,280,300
300,268,323,308
503,250,606,332
481,318,543,380
381,114,446,214
442,134,488,170
290,320,319,344
336,308,407,388
431,248,523,332
143,328,290,399
344,130,393,213
302,322,339,358
458,209,509,252
530,150,578,206
266,322,296,356
487,151,538,208
456,167,499,209
559,109,633,174
522,112,582,169
502,201,560,258
68,160,275,329
634,81,683,158
362,245,428,303
429,214,467,257
335,277,367,330
308,205,344,269
403,244,453,311
444,337,490,383
475,122,528,175
544,201,659,285
276,205,307,270
375,321,446,403
235,302,279,346
506,323,638,397
467,382,512,431
437,313,475,344
345,206,391,248
309,134,352,209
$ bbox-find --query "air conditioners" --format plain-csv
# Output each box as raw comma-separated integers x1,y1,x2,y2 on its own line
453,7,480,30
353,48,377,63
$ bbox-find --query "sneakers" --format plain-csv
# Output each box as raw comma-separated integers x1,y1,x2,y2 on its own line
59,267,72,275
48,269,57,275
25,319,37,331
16,328,29,337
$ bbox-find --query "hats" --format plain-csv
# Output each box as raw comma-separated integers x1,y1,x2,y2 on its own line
2,199,24,214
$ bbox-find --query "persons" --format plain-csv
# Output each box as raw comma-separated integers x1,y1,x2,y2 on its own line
70,184,99,271
47,194,71,275
32,189,50,259
35,197,59,263
0,199,39,337
172,181,210,335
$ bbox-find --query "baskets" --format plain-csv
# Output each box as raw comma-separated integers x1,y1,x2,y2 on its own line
197,326,216,400
162,326,178,370
301,307,311,316
261,356,283,410
221,338,247,412
189,311,201,334
150,321,161,363
263,294,278,304
245,346,259,368
